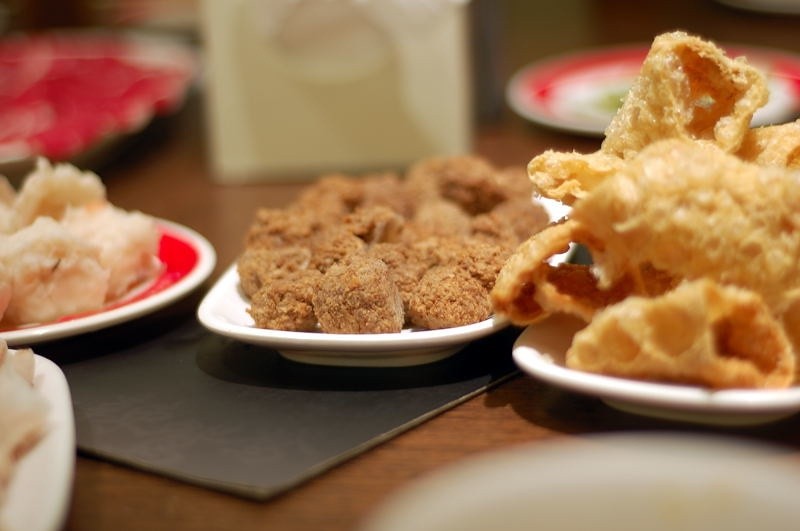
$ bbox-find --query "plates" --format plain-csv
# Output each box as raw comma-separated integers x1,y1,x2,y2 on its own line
1,51,196,189
1,350,78,531
505,43,799,140
197,197,579,369
512,320,798,428
0,215,218,345
